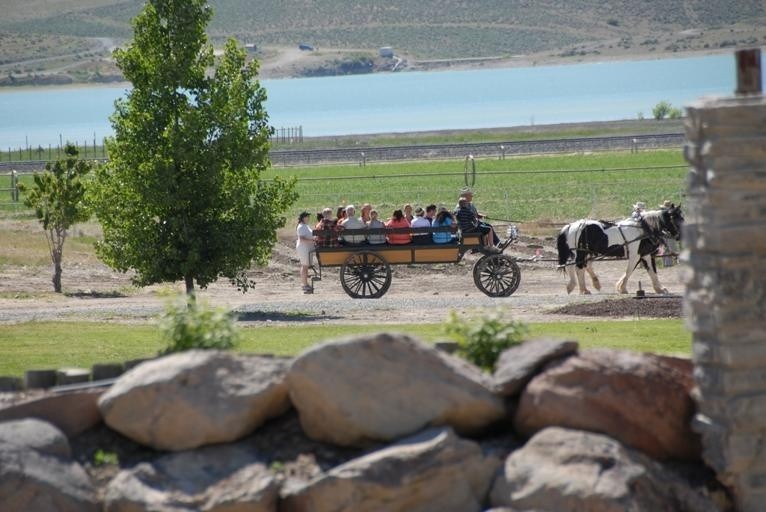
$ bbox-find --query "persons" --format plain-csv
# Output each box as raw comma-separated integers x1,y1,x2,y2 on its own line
459,186,503,249
294,209,318,293
456,197,503,256
311,202,460,249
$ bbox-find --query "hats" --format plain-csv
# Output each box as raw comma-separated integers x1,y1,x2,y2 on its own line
658,200,672,209
298,210,311,221
460,187,473,197
415,206,425,216
632,201,647,209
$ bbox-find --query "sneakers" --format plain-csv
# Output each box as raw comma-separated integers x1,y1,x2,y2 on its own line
301,285,315,290
484,244,490,251
489,244,503,252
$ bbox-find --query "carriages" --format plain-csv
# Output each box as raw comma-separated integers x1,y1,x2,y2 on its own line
302,201,687,301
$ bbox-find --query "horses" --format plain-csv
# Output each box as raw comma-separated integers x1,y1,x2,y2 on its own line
555,202,685,296
